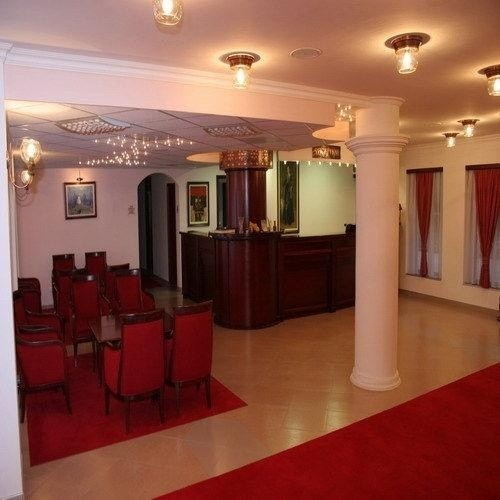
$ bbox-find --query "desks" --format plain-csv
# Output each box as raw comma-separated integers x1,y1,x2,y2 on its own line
85,308,174,387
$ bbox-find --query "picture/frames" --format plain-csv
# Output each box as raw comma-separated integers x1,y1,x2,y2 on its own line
277,160,300,234
186,181,210,227
215,174,227,230
63,181,97,220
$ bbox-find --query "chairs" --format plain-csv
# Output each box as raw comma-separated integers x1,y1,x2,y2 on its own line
17,277,43,313
57,275,74,339
162,300,214,415
12,290,73,424
112,268,156,314
62,274,111,368
85,251,106,270
52,254,76,270
103,263,130,300
96,309,167,435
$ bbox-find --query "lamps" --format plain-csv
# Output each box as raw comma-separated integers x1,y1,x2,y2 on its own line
457,119,480,138
152,0,184,26
476,64,500,96
384,32,431,74
8,136,42,189
219,51,261,89
442,133,460,148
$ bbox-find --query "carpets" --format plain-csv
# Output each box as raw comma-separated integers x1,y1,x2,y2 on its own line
216,362,500,475
26,352,249,466
